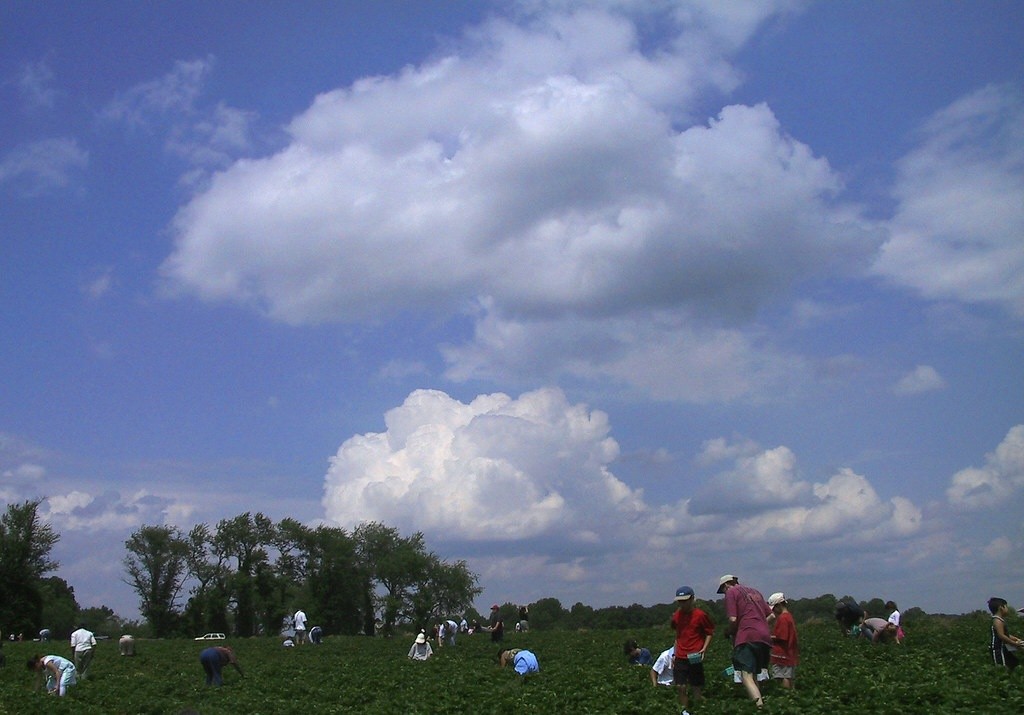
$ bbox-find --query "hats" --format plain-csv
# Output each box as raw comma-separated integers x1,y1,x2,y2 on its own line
673,586,696,601
833,601,847,615
415,632,426,644
490,604,498,609
767,593,788,610
716,575,738,594
886,622,899,637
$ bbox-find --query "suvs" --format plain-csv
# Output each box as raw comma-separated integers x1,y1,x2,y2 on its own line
194,632,226,641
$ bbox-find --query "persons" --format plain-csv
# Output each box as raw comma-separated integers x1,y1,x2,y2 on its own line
650,646,675,686
670,585,712,715
501,649,539,675
432,625,438,640
8,631,23,640
834,601,905,644
439,620,458,647
293,609,308,644
309,626,322,644
71,623,97,675
458,617,482,634
408,633,433,661
488,605,503,643
623,640,652,665
27,655,76,697
38,629,51,642
716,575,800,711
519,606,529,632
119,634,135,657
201,643,243,686
988,597,1021,668
283,637,295,647
